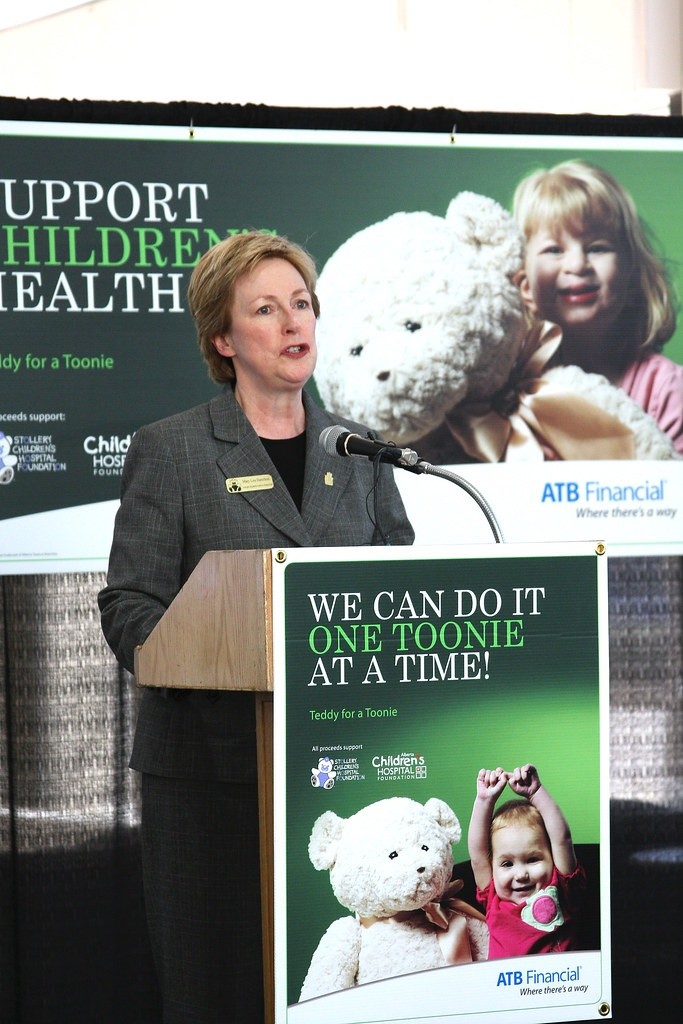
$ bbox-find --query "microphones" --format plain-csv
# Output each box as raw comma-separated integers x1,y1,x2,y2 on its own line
366,430,375,441
319,425,417,466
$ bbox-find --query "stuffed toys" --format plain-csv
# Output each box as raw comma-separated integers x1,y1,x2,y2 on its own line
309,192,679,462
297,796,489,1001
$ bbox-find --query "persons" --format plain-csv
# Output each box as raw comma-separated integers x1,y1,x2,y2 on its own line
99,233,415,1024
468,764,588,960
507,157,683,457
